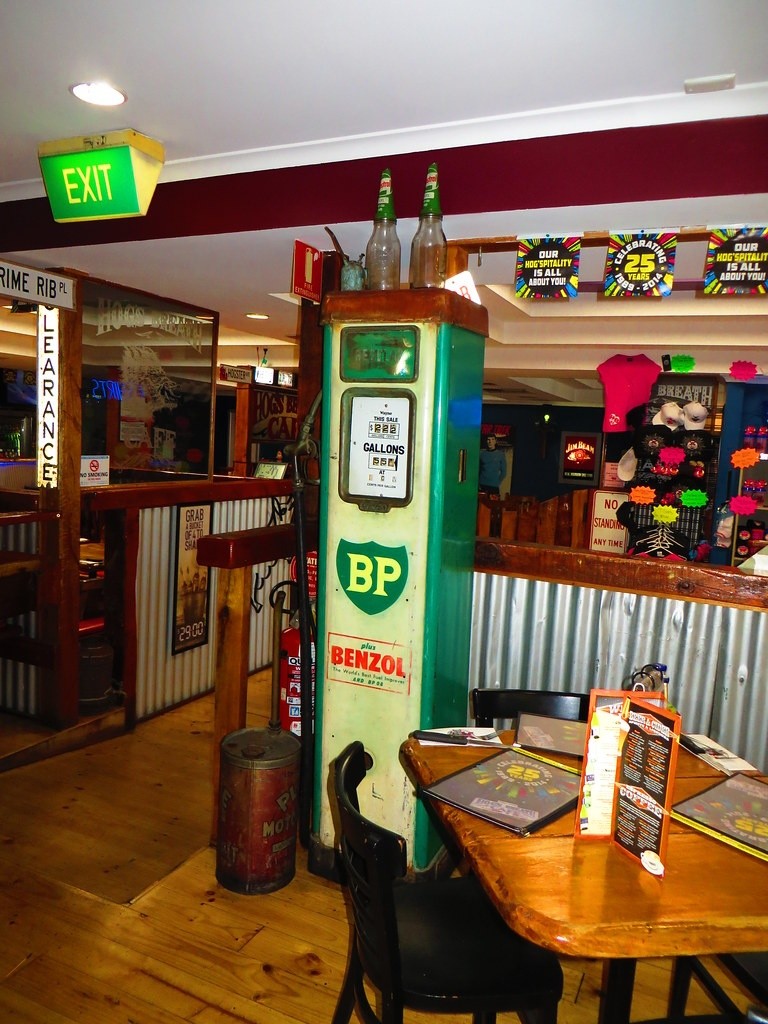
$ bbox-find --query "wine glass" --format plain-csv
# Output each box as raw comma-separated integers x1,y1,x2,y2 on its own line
4,448,18,462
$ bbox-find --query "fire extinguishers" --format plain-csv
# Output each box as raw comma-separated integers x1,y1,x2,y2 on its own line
270,579,316,740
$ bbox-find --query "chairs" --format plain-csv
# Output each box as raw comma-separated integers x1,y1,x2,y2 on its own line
471,688,590,728
330,741,563,1024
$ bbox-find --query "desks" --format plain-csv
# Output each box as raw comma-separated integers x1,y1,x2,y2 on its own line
398,728,768,1024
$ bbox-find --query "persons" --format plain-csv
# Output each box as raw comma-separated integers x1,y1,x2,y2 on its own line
478,434,506,495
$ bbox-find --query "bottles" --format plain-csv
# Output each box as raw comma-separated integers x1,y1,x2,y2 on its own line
409,162,448,288
365,168,400,291
340,261,365,291
0,426,21,456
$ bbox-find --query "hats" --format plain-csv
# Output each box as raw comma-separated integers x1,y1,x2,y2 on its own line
651,401,684,431
683,401,708,431
617,446,638,482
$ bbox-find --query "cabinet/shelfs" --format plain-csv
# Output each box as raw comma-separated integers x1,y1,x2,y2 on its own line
628,373,768,577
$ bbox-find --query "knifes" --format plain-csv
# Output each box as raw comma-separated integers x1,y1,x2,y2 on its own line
412,730,505,746
